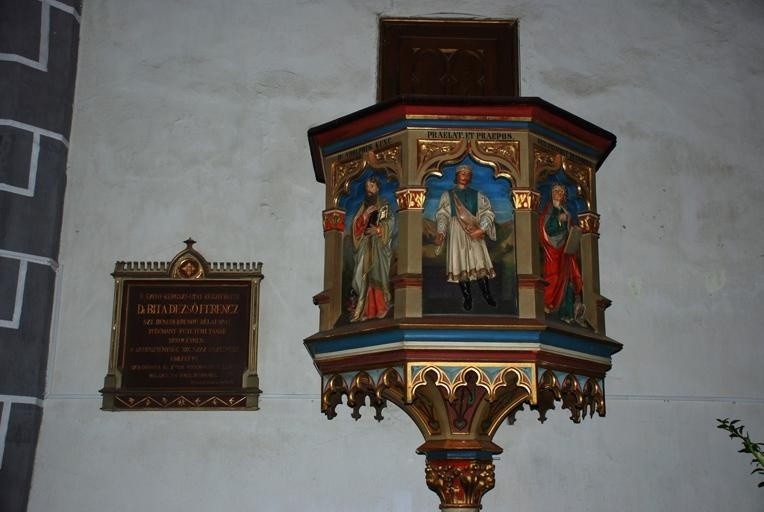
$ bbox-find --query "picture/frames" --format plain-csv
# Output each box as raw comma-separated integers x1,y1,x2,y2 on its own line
98,237,263,411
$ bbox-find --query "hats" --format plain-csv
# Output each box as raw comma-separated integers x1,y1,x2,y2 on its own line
455,165,474,173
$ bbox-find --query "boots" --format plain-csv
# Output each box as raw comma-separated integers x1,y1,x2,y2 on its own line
479,277,497,306
460,278,473,311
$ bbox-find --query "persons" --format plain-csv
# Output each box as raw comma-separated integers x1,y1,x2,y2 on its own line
431,164,499,311
349,175,396,324
538,182,584,323
